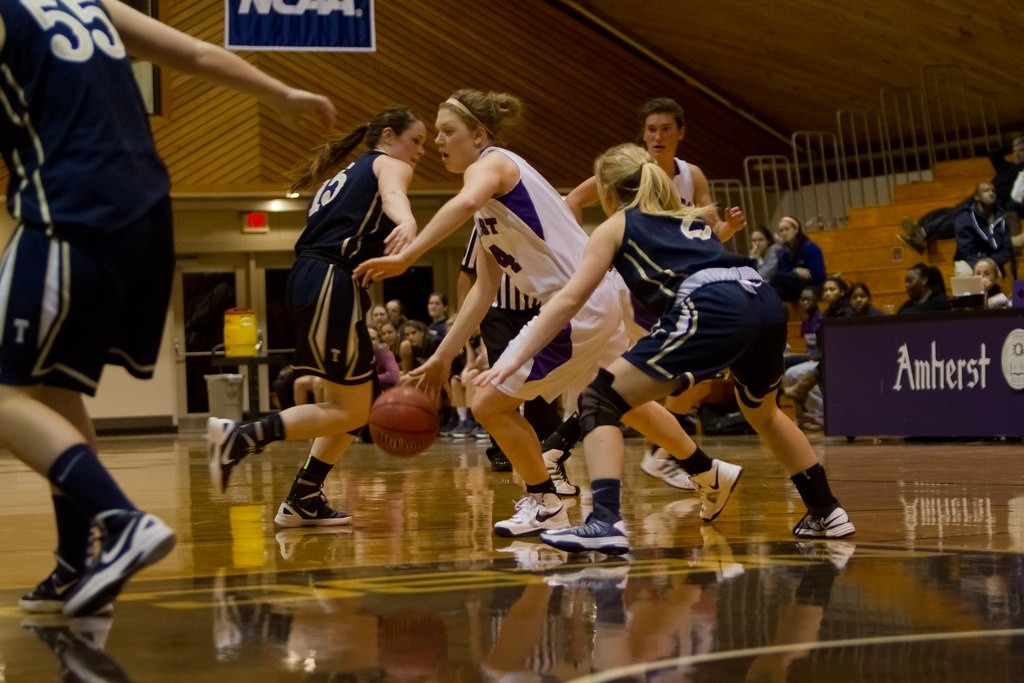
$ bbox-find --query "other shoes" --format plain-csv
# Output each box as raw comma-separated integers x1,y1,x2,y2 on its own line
902,217,930,248
490,444,511,471
898,235,926,254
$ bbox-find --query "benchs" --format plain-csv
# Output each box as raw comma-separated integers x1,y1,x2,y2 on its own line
705,158,1024,387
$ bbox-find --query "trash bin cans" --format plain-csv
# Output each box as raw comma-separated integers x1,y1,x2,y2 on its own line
204,374,245,424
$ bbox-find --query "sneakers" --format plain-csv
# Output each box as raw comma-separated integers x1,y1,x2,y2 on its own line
542,449,584,497
19,550,112,614
206,416,263,494
642,446,696,491
543,513,630,556
495,497,572,536
274,489,353,527
690,456,744,522
65,510,177,617
439,420,492,439
792,506,857,539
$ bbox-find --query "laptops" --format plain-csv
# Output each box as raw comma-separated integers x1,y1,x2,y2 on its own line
950,275,985,297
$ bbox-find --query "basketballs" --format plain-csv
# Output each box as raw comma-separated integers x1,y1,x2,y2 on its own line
368,385,441,457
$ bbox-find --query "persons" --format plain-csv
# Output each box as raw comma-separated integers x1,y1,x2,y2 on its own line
536,98,747,497
897,262,953,313
974,256,1004,311
457,224,575,472
748,216,827,303
367,292,492,439
781,278,884,431
351,88,744,537
471,142,856,554
898,136,1024,255
209,104,427,527
951,167,1024,281
0,1,338,616
